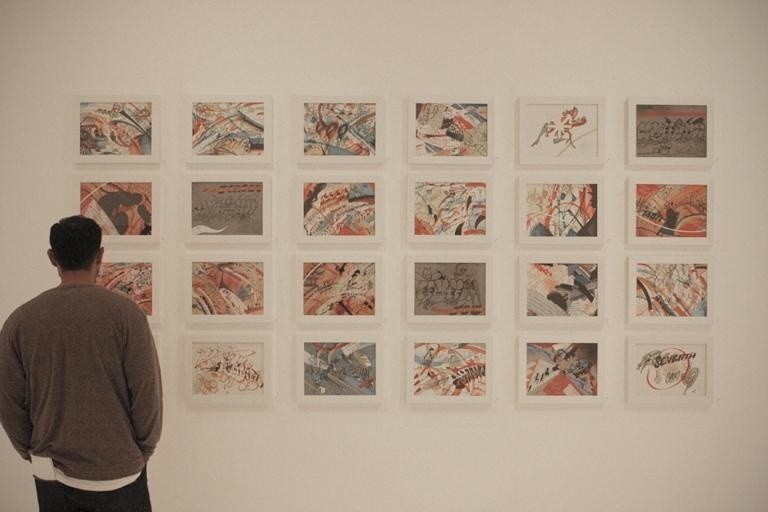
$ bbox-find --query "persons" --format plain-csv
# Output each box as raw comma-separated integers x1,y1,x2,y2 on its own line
0,216,163,512
